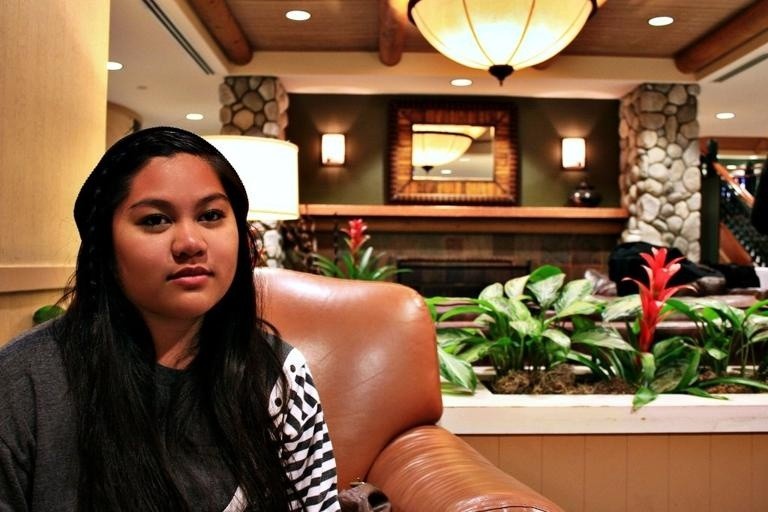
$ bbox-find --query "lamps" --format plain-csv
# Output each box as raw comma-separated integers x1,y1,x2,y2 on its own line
320,131,347,168
406,0,607,88
201,135,299,265
559,137,587,170
410,123,488,173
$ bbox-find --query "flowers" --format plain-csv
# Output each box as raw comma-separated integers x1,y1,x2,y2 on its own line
603,243,702,388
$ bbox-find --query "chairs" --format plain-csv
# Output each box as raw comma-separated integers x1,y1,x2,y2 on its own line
251,262,562,512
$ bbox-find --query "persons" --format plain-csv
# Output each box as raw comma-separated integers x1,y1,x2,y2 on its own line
0,126,341,511
745,161,757,196
751,155,768,236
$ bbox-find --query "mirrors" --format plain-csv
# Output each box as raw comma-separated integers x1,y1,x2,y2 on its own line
384,101,523,206
